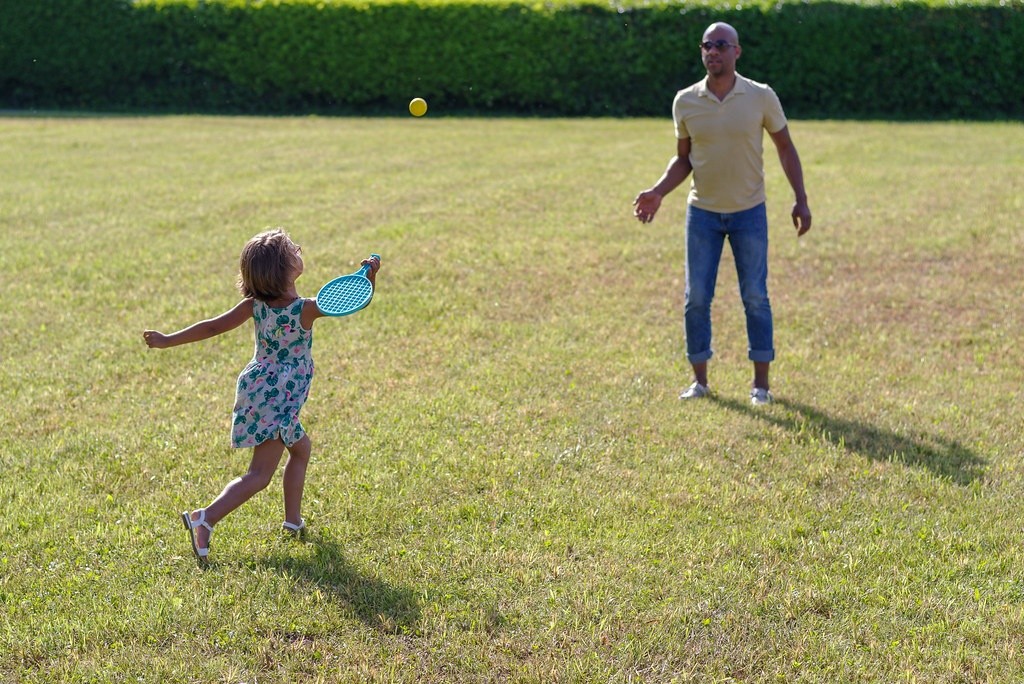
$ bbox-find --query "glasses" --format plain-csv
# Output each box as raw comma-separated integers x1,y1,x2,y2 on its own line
697,40,739,50
293,244,302,256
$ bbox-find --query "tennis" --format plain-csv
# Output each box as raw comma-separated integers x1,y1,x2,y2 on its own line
409,99,426,115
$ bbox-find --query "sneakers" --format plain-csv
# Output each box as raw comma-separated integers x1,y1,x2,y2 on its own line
678,379,708,402
751,386,771,404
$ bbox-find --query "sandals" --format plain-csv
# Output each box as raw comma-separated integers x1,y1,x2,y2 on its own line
280,520,306,540
182,509,213,567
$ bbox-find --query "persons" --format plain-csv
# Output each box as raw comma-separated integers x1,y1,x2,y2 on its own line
631,22,811,408
143,230,381,570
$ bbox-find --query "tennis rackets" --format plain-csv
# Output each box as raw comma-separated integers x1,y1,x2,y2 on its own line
313,256,381,312
